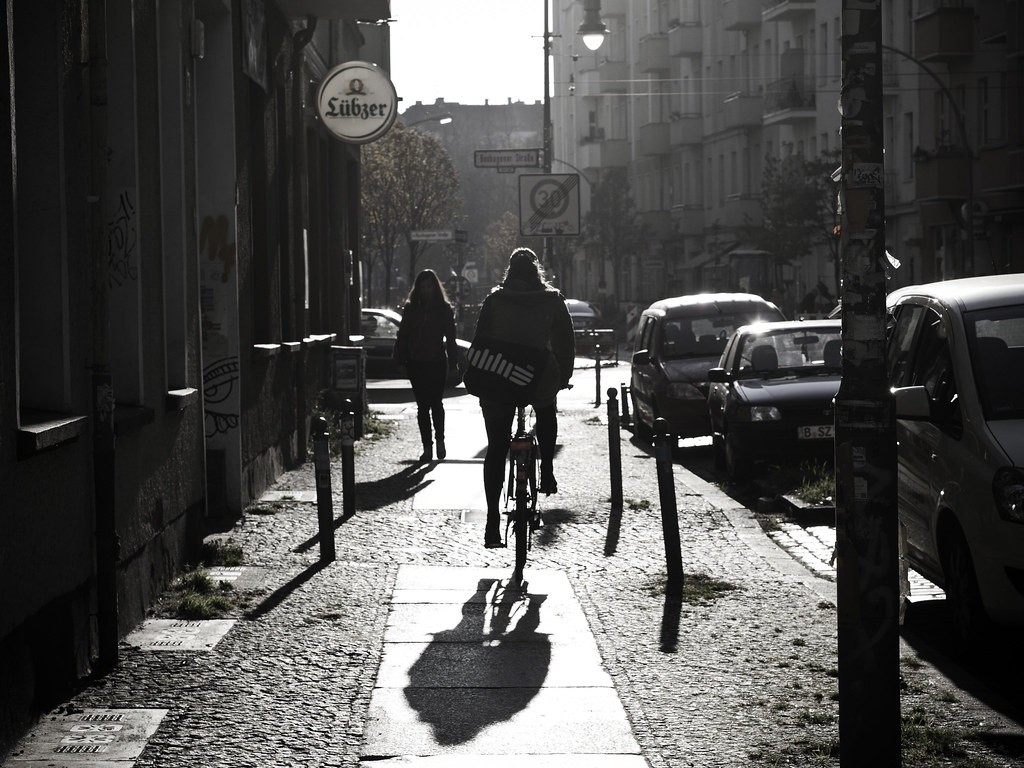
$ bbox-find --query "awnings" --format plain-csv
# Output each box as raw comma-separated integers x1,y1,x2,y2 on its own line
676,241,737,269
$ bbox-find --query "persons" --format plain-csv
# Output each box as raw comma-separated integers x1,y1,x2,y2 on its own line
390,269,458,461
799,282,834,314
463,250,575,544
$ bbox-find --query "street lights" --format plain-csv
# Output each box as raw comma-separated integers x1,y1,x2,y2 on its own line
838,34,977,276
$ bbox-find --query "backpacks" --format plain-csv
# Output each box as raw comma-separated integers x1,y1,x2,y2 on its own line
480,286,562,401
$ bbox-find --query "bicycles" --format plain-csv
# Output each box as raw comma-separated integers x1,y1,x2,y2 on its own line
506,385,572,569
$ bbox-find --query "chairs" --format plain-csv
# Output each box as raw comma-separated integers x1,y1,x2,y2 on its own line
675,328,695,344
823,339,842,366
977,335,1024,408
751,345,778,371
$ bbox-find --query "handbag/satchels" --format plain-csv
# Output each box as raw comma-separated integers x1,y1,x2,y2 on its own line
391,340,409,379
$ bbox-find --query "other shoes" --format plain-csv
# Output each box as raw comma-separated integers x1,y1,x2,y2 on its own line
437,440,446,459
540,474,558,493
484,526,501,545
420,452,433,461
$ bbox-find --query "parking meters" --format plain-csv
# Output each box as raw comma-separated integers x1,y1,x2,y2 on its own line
330,345,367,516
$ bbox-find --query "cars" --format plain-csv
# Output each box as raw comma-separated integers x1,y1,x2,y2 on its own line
708,319,843,498
565,299,615,355
362,308,474,387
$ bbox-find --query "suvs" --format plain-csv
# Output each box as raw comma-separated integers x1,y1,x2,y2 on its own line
884,272,1024,630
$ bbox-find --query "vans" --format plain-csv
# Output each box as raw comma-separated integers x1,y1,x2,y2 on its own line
631,294,794,449
